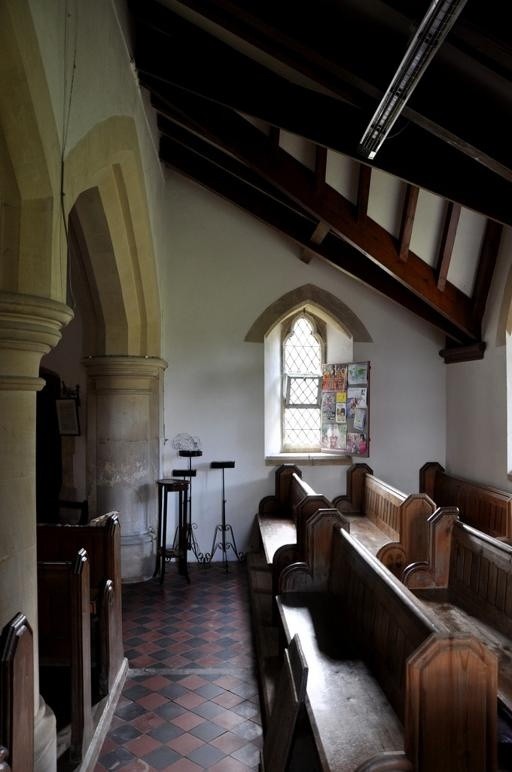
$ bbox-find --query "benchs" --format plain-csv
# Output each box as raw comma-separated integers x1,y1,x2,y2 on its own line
42,546,102,762
32,510,123,694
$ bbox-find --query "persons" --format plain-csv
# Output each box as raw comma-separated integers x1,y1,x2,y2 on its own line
356,433,366,455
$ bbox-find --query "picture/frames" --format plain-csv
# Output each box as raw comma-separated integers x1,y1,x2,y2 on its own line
55,397,81,436
